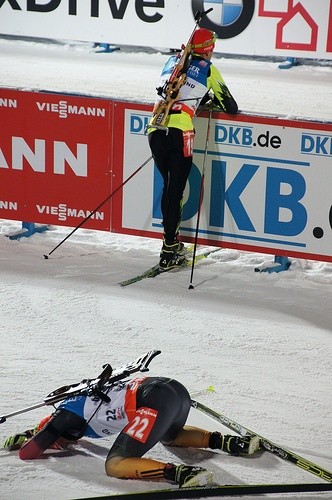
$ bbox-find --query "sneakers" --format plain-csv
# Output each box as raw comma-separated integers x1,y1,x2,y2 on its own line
219,433,259,456
160,249,186,271
175,464,212,488
161,239,184,254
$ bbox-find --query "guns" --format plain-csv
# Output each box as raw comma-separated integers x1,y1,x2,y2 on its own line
0,350,162,424
147,8,213,129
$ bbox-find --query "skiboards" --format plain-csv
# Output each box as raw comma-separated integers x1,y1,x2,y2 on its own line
117,244,224,287
70,483,332,500
190,400,332,482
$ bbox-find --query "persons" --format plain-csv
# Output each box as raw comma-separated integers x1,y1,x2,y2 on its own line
146,28,237,272
5,378,262,488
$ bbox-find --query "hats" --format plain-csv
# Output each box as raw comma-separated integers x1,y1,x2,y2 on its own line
39,414,63,450
189,29,215,53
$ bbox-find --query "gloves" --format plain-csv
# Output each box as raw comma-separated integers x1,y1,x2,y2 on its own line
4,426,38,450
